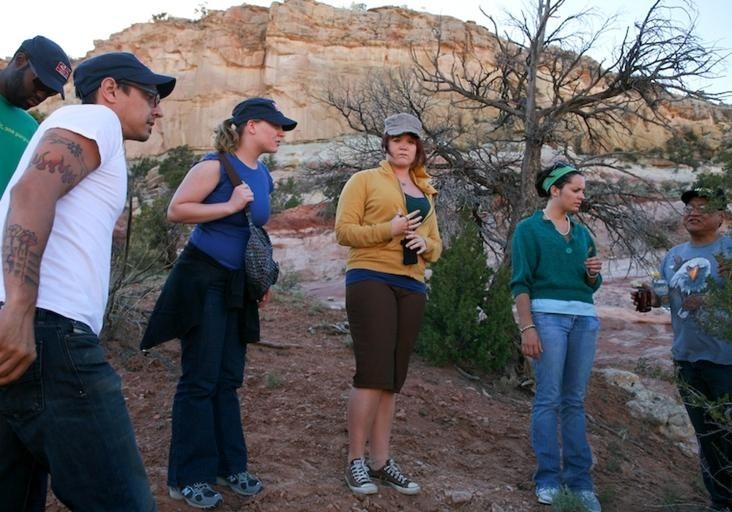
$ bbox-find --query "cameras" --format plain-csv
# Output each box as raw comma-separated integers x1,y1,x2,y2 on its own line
635,286,654,313
399,238,418,265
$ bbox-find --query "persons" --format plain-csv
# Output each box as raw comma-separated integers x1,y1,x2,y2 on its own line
334,112,446,499
506,163,606,512
139,96,298,512
0,51,164,511
630,179,729,511
0,34,70,194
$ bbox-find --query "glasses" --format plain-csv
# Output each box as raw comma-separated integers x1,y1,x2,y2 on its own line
117,79,160,107
683,205,708,213
28,57,59,96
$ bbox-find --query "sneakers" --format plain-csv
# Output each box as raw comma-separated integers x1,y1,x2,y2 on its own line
344,457,379,494
564,482,601,512
216,470,265,496
534,484,562,505
169,481,224,508
366,458,420,495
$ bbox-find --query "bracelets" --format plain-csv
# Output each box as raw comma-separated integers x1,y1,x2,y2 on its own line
654,294,662,308
519,322,536,334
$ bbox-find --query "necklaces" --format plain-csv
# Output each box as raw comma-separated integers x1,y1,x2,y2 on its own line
394,172,411,185
542,209,570,236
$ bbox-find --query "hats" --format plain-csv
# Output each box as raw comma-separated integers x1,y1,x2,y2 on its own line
232,98,297,131
22,35,72,100
384,112,423,138
681,187,726,211
73,52,176,99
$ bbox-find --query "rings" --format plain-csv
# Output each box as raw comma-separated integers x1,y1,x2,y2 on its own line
397,213,411,236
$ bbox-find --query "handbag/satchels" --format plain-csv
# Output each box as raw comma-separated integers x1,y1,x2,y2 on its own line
245,225,278,302
